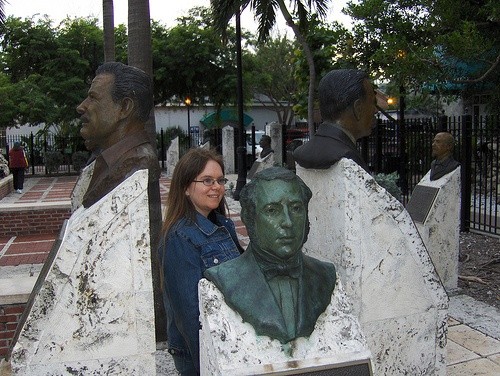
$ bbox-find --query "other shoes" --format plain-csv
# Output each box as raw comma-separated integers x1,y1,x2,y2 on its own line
17,189,22,194
14,189,17,193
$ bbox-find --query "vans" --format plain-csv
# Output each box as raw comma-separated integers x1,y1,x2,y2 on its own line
242,129,266,168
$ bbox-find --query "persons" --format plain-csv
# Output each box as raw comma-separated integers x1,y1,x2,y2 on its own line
77,60,152,208
294,69,377,170
259,135,274,158
9,142,29,194
203,167,336,344
157,148,245,376
429,133,459,181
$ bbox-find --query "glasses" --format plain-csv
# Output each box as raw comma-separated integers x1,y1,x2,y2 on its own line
191,178,229,186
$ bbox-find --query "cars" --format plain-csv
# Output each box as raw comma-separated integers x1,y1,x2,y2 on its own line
284,139,309,165
